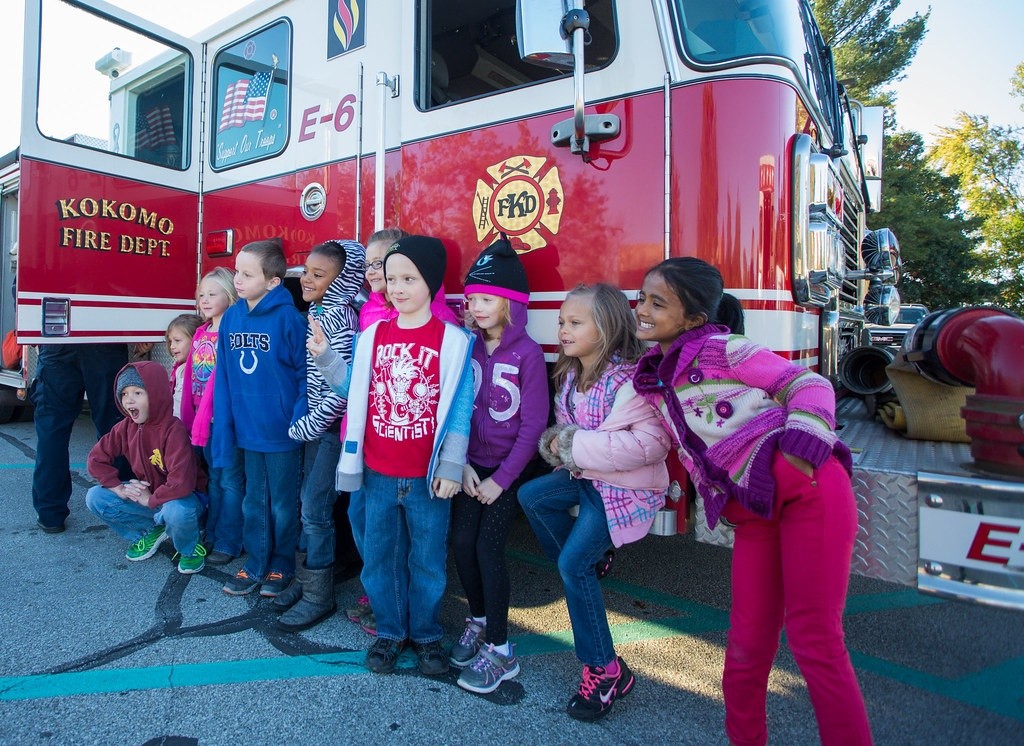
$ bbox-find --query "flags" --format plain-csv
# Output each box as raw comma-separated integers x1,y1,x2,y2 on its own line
217,68,274,128
122,97,178,148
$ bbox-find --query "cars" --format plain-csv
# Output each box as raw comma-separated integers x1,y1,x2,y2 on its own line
863,304,929,349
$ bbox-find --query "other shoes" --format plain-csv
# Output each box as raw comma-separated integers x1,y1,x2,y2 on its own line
208,550,233,564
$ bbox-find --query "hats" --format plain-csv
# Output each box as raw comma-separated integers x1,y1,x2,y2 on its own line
464,240,530,304
383,237,447,303
117,367,146,405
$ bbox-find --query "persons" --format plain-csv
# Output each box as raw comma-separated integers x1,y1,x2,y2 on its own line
84,362,210,574
11,272,154,535
305,234,477,679
631,255,876,746
175,262,245,569
166,312,204,435
266,238,367,635
448,240,550,697
338,225,459,637
209,235,310,597
517,281,670,722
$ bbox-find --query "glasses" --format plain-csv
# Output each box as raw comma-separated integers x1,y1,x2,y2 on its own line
362,261,383,272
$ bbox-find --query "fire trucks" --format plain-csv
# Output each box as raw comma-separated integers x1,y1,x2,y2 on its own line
0,0,1023,613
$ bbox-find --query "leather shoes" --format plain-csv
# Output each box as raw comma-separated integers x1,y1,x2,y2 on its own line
37,515,65,533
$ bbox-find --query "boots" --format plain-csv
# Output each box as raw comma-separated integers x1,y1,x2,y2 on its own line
268,550,308,612
275,558,337,632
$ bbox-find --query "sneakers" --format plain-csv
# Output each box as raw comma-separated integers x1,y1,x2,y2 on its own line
593,548,614,580
451,617,487,666
410,637,449,673
260,571,291,596
346,595,372,621
177,530,205,573
457,642,520,693
360,612,379,635
368,638,407,672
126,525,169,562
222,570,258,595
569,656,635,720
